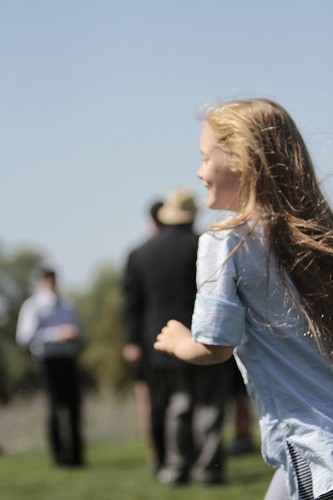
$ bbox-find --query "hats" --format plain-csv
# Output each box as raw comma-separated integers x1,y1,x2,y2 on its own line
156,188,197,225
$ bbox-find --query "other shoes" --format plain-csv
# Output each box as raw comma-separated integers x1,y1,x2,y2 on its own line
158,469,186,485
186,465,220,483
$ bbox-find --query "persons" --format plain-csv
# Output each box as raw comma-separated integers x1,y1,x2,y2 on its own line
228,364,253,456
120,187,229,486
153,97,333,500
121,201,169,474
15,268,87,470
